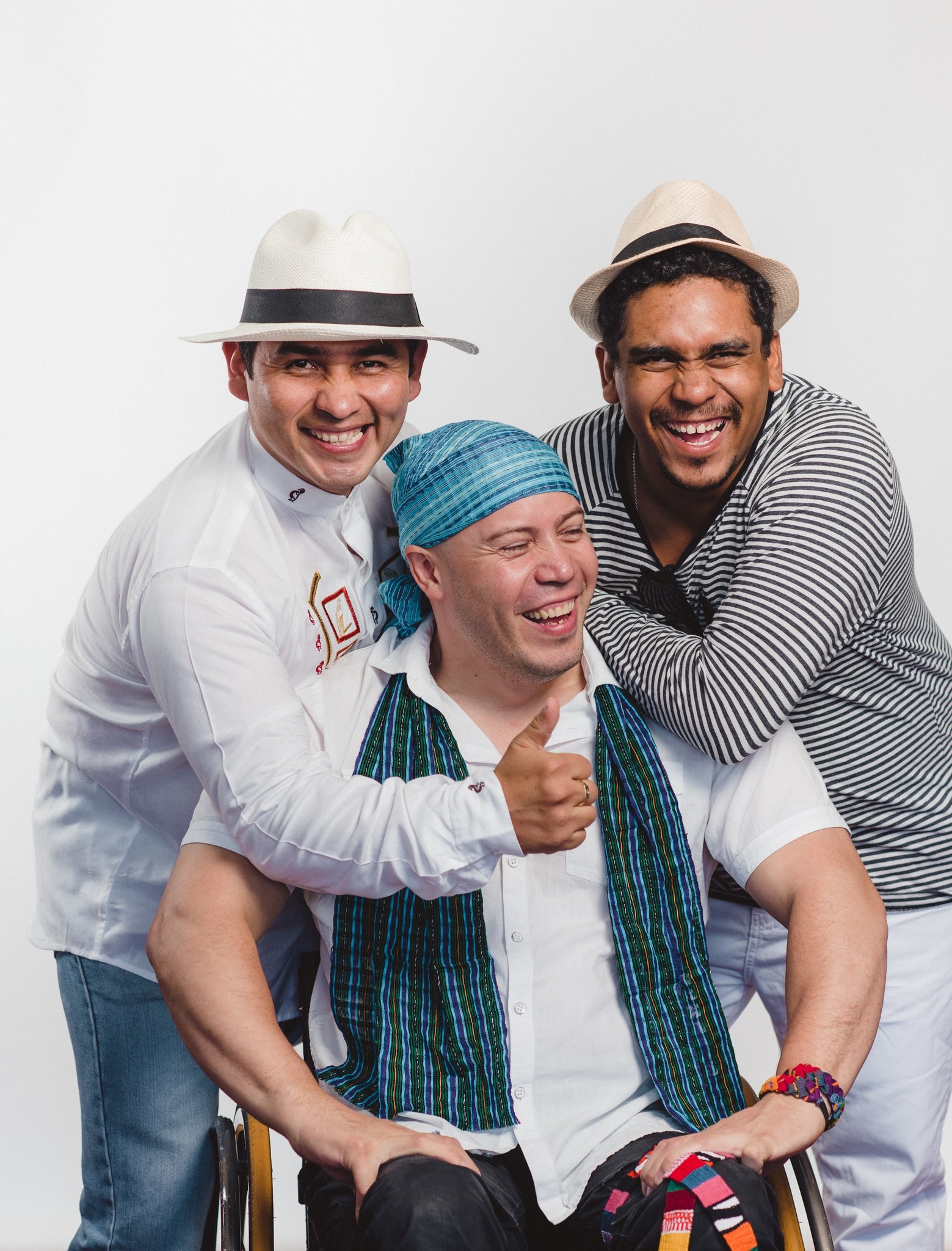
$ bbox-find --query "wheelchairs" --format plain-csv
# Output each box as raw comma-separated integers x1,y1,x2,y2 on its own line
204,1014,838,1250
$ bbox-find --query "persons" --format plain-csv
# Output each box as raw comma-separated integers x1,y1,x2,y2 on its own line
145,419,891,1251
31,207,599,1251
542,180,952,1251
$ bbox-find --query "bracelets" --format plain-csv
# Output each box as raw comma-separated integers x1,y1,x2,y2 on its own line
755,1061,844,1129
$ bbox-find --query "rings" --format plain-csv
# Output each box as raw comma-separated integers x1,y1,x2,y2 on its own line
577,777,589,807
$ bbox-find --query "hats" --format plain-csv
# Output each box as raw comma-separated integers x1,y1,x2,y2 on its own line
178,208,481,357
567,178,801,342
